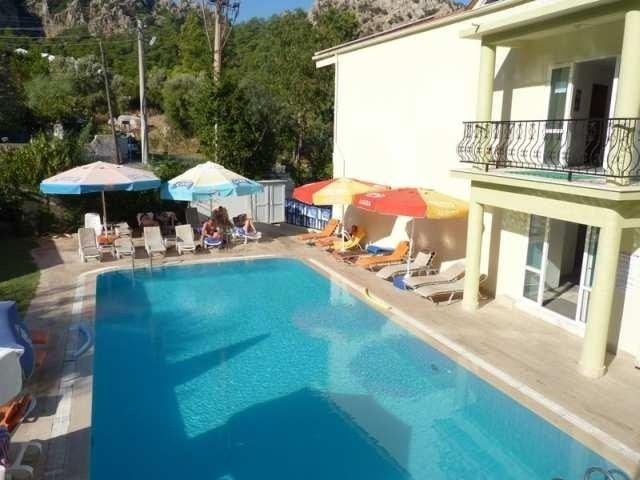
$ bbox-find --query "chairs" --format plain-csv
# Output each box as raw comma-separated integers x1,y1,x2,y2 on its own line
296,218,489,306
0,392,44,479
77,211,262,264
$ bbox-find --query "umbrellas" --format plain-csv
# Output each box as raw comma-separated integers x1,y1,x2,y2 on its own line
0,300,35,406
292,177,391,242
350,335,456,399
40,161,161,240
295,300,388,344
160,160,264,215
352,188,469,274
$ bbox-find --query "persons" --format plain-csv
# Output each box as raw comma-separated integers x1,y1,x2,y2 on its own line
315,225,357,247
140,207,256,251
